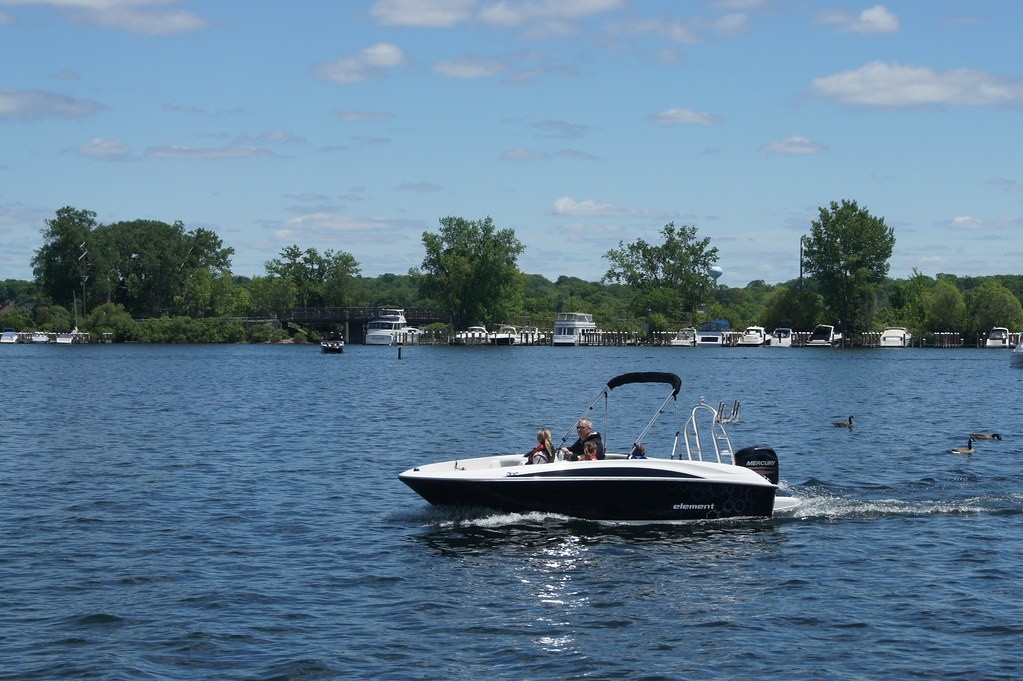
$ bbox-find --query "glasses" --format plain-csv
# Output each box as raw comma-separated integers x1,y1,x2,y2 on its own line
577,426,589,429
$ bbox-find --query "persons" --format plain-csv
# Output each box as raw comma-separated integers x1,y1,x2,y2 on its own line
558,417,605,462
524,429,555,465
578,441,598,462
628,442,648,459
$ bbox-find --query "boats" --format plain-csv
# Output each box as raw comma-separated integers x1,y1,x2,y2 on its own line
670,328,732,346
398,371,780,519
454,325,547,345
735,324,794,348
879,327,911,347
985,327,1012,348
321,331,345,353
1,327,115,345
365,309,422,345
549,311,597,344
